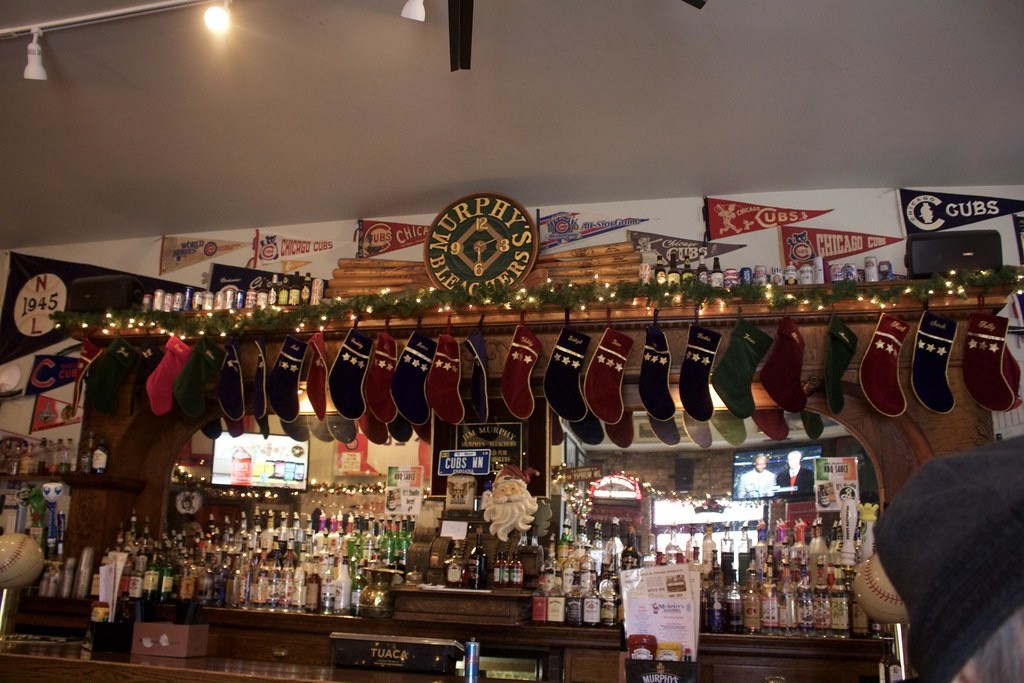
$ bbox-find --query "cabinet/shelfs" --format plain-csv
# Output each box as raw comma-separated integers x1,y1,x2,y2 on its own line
113,596,893,683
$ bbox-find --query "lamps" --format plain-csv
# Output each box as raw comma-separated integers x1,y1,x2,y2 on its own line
402,0,426,21
21,26,51,82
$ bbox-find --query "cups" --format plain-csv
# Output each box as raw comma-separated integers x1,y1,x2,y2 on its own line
39,547,95,598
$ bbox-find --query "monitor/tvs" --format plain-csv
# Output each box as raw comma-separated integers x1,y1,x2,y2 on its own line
210,430,310,493
731,442,822,501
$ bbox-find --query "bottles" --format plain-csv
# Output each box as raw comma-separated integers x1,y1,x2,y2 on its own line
696,253,708,284
467,528,488,590
0,432,109,475
300,272,312,305
799,264,814,284
90,507,414,622
231,445,253,486
443,541,467,589
521,532,527,547
667,254,680,286
710,258,724,288
493,550,509,588
279,274,290,306
819,485,830,508
879,632,902,683
288,271,301,306
268,272,279,305
530,518,896,637
655,255,667,287
784,261,798,285
509,550,524,588
465,638,480,683
682,256,694,286
684,648,691,662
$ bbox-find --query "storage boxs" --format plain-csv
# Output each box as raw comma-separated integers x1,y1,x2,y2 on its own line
131,622,209,658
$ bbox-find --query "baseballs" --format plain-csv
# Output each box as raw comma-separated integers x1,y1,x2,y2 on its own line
854,552,910,624
0,533,45,590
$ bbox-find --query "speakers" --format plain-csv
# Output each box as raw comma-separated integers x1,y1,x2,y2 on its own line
675,458,694,494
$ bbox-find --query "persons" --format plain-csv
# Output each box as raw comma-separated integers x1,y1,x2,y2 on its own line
490,465,528,520
776,449,814,496
736,453,776,497
